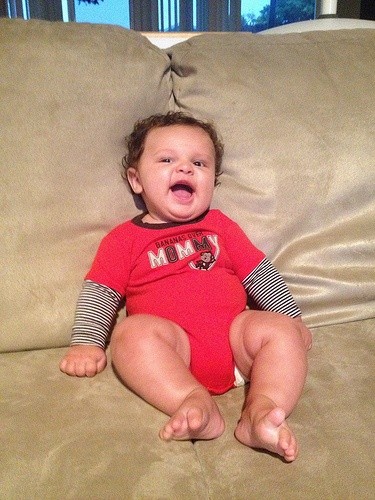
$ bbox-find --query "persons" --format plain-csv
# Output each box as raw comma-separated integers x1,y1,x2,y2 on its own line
59,110,313,463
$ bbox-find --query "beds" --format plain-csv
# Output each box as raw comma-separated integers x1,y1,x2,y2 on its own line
1,16,375,500
